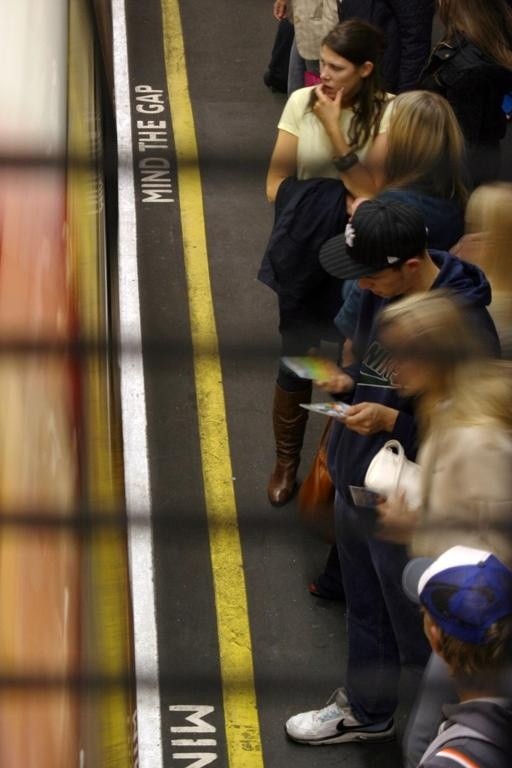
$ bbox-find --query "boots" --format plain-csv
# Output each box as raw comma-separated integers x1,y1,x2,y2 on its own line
267,380,312,506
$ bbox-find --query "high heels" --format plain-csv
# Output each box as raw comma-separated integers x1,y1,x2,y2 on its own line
265,71,279,94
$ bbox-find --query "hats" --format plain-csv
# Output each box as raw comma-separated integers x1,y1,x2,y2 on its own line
319,198,430,279
400,545,511,645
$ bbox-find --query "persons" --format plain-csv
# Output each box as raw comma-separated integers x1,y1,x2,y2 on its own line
264,1,512,768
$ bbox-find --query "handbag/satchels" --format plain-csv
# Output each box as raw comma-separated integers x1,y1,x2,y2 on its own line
364,440,424,511
418,33,506,145
297,448,334,542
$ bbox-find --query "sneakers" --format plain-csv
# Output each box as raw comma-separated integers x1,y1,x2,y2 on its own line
284,686,397,745
